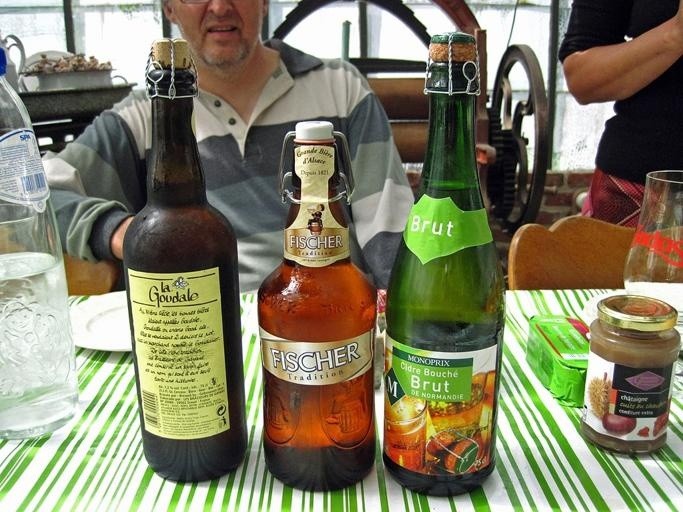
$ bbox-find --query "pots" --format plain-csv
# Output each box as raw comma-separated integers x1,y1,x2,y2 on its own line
36,68,128,90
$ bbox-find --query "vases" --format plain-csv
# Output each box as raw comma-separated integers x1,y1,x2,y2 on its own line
38,71,113,90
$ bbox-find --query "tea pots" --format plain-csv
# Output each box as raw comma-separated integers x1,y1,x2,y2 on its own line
0,35,26,91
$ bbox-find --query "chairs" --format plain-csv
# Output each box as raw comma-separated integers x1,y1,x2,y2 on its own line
507,215,683,290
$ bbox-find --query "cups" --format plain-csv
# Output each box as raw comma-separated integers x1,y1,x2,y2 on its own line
623,171,682,377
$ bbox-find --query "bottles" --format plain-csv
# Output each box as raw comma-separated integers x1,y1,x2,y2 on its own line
256,121,376,490
386,32,505,497
0,49,77,439
120,40,247,482
375,290,388,400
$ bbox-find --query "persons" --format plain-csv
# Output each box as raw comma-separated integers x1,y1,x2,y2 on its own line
559,0,683,229
11,1,418,293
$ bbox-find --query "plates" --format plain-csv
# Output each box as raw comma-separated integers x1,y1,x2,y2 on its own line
584,294,608,327
72,290,135,352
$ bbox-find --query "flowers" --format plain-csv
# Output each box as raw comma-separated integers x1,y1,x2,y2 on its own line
20,52,113,75
